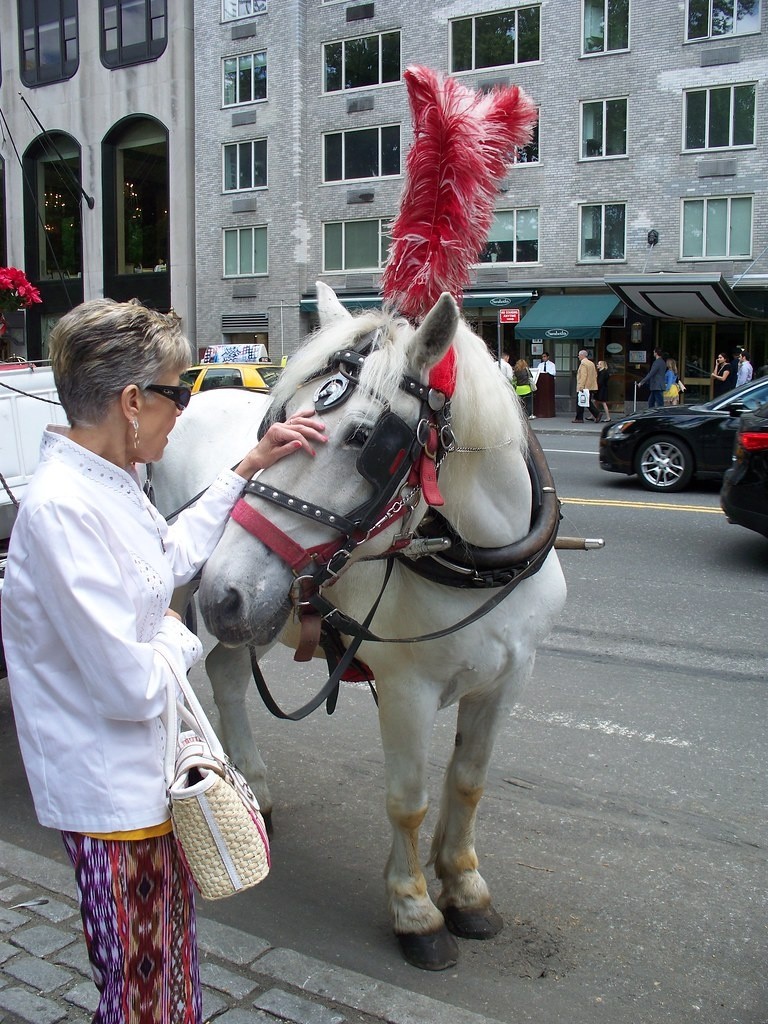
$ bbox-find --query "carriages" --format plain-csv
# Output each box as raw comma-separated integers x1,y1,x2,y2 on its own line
0,280,604,968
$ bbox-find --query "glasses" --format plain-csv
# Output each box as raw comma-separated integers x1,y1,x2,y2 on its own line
136,384,192,411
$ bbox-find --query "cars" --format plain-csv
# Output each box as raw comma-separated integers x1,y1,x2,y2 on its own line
178,343,291,395
599,374,768,489
718,401,768,539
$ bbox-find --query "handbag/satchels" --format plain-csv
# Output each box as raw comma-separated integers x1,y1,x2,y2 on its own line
676,378,687,394
525,366,537,391
146,642,271,902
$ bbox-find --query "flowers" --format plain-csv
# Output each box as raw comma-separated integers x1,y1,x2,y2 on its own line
0,267,42,334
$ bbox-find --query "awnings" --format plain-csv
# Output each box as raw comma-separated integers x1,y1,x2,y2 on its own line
514,293,620,340
604,272,768,324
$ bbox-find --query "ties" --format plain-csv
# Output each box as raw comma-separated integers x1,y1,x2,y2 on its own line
543,362,546,372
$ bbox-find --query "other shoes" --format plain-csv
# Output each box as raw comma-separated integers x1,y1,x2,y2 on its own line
528,416,534,420
572,419,584,423
585,417,593,421
593,413,603,423
601,419,611,423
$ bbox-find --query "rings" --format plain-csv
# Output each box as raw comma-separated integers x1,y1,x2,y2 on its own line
289,418,294,426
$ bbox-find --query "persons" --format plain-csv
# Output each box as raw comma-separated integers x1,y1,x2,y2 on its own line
711,347,753,398
572,349,611,423
494,352,556,419
0,296,327,1024
637,348,680,408
